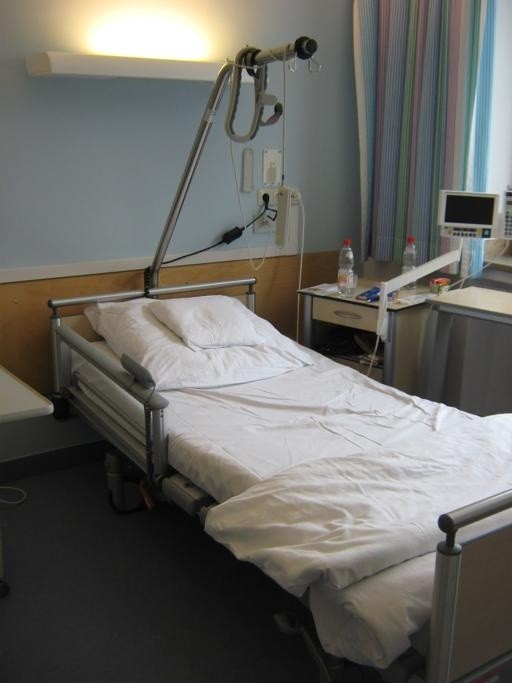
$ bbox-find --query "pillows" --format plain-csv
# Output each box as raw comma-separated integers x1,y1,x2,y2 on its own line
146,294,267,351
80,298,315,393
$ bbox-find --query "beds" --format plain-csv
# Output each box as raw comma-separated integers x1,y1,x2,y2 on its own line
46,276,512,683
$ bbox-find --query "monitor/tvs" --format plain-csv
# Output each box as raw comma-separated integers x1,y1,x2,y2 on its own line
437,190,500,229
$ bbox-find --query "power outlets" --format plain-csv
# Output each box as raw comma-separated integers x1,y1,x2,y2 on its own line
257,188,275,207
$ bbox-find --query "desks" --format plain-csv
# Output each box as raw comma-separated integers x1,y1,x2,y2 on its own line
2,364,55,604
418,284,512,410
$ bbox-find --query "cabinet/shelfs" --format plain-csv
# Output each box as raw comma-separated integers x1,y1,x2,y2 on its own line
296,278,439,397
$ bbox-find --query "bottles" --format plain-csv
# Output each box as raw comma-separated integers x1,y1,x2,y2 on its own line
401,236,417,289
336,238,353,293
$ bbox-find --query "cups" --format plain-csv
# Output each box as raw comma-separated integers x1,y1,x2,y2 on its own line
345,274,357,297
